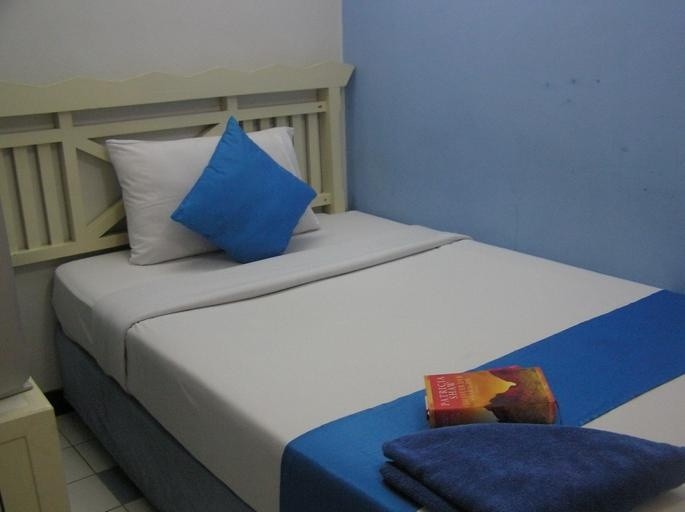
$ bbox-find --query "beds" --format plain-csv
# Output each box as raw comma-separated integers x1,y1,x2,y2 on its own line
47,204,685,510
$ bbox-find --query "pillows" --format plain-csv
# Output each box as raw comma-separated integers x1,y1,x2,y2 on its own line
106,117,322,263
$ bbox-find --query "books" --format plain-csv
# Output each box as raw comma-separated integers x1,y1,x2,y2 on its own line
424,364,557,429
